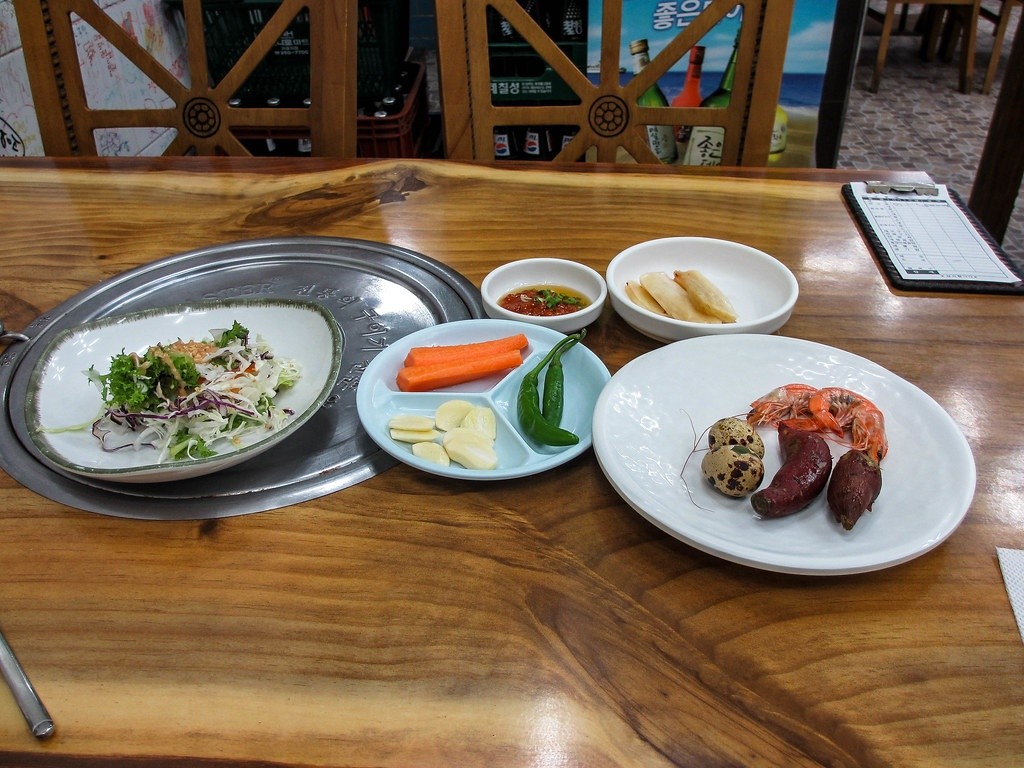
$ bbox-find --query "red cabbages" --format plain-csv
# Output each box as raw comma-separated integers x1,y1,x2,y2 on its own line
91,387,255,451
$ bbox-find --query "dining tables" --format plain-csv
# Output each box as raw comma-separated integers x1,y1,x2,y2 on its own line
0,155,1024,768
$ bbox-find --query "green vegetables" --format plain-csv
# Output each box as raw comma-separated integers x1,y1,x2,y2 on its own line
99,348,198,413
171,429,214,458
211,322,248,368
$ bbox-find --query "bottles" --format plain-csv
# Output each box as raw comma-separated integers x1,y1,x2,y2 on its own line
680,27,746,166
215,67,412,158
629,38,679,164
491,125,581,160
496,0,583,77
766,104,788,161
671,45,707,142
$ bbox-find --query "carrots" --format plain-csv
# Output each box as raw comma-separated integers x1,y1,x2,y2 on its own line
405,333,527,371
396,350,523,391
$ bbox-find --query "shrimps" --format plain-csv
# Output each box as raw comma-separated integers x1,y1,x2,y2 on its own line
809,387,889,467
746,383,828,431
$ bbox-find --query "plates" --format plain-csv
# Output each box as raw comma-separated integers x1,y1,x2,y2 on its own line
356,318,612,479
591,333,978,577
480,257,605,331
23,296,342,485
606,237,800,340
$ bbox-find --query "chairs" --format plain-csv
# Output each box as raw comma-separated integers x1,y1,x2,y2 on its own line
14,0,359,156
435,0,794,167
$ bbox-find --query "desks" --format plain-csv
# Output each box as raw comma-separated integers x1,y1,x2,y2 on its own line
862,0,1024,95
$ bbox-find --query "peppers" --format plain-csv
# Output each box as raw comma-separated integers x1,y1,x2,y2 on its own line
518,333,580,446
543,328,586,427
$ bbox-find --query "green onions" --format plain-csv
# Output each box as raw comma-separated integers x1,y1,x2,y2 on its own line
536,289,576,307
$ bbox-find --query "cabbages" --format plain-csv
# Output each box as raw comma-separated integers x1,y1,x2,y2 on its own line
187,327,304,448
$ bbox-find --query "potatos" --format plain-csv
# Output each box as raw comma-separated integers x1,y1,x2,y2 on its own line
389,401,498,470
751,422,832,518
828,448,882,529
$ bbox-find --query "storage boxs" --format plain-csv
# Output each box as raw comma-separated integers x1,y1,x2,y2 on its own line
172,0,411,100
214,61,431,158
426,45,588,115
